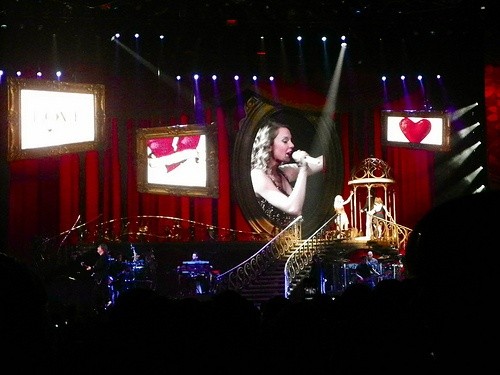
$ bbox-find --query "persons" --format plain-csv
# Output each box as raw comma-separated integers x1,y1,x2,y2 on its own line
0,253,500,375
79,243,113,312
356,250,380,288
406,149,500,334
370,197,391,238
250,120,324,228
191,252,201,262
334,191,354,231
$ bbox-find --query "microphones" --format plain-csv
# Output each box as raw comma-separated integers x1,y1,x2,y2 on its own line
292,151,322,165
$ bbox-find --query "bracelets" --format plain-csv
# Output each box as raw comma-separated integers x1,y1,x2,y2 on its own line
296,161,308,167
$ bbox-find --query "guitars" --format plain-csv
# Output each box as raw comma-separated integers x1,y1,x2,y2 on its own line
357,270,393,285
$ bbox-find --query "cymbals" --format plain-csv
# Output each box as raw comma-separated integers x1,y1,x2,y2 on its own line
332,259,352,262
376,254,394,260
